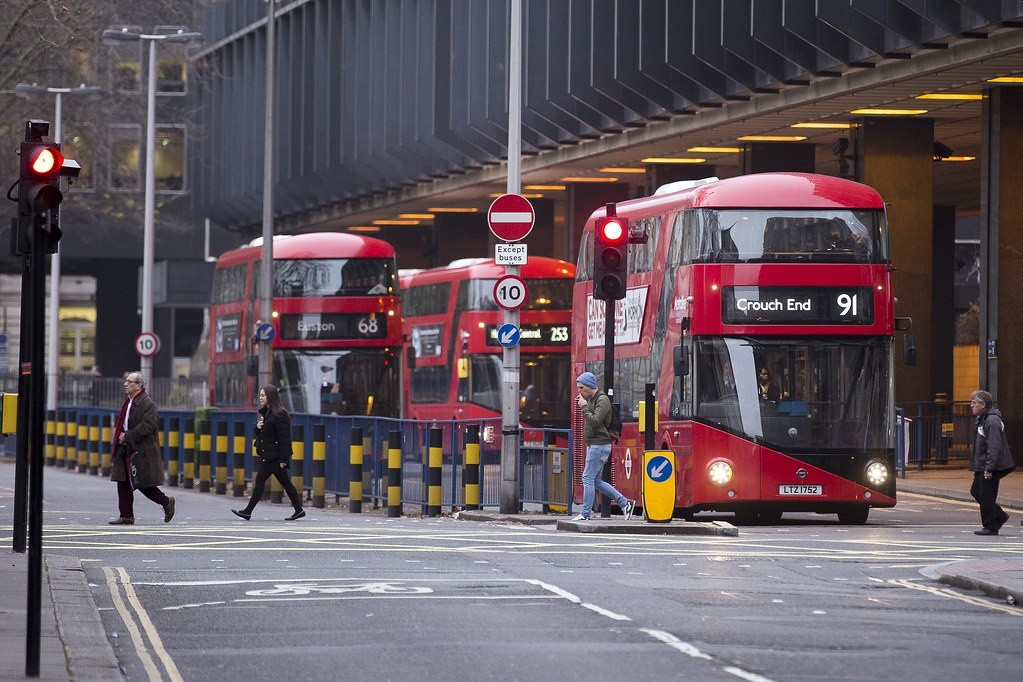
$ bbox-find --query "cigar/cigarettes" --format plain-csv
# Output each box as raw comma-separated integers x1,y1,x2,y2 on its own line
577,395,580,398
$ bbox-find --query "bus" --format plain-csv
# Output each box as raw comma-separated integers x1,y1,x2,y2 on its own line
206,231,401,473
392,256,577,466
570,171,917,528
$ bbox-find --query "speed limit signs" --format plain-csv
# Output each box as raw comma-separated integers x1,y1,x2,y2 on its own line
493,274,530,312
135,332,159,359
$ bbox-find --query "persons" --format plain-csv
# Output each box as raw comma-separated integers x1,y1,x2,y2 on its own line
706,358,823,405
571,372,636,521
970,390,1015,535
89,367,106,407
232,385,306,521
108,374,175,525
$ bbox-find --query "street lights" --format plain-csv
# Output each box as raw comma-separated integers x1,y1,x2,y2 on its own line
99,26,205,397
16,80,102,408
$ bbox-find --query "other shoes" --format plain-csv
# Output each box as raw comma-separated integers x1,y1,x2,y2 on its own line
232,508,252,521
975,527,999,536
998,512,1010,530
285,511,306,520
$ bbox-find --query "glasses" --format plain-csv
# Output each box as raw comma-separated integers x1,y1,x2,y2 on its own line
125,379,137,385
761,372,768,376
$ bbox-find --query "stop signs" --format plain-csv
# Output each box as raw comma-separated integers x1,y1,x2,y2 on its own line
488,193,535,242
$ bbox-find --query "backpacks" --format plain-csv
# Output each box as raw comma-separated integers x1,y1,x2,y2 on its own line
594,394,623,445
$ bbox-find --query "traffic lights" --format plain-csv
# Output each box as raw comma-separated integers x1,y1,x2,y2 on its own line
18,140,64,254
594,213,629,300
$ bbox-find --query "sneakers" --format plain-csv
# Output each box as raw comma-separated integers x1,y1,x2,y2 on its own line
621,498,636,520
164,497,175,522
108,516,135,525
572,515,588,520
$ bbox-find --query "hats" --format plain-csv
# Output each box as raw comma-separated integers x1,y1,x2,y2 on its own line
577,372,597,389
